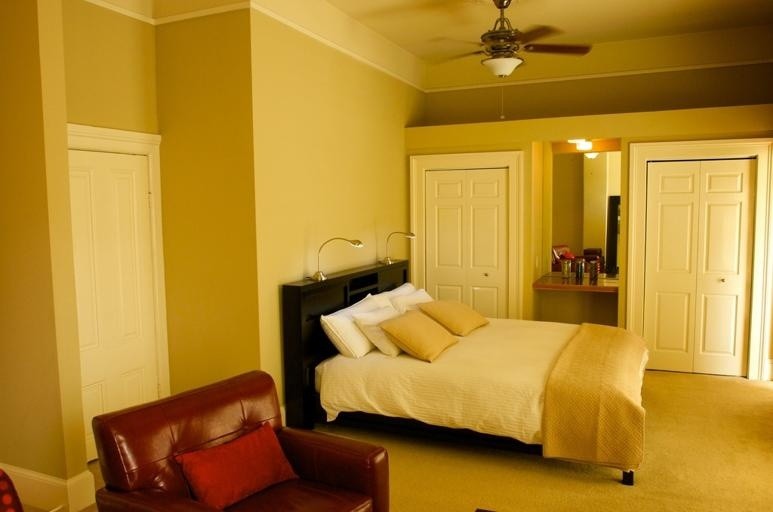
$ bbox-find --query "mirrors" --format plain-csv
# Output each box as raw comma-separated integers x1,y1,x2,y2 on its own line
552,152,621,271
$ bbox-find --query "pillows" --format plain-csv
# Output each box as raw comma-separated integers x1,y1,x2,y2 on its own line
354,307,403,356
175,422,297,510
392,288,432,312
320,293,377,358
381,283,416,311
419,300,488,336
380,311,458,363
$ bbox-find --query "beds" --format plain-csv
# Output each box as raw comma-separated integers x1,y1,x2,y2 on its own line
283,259,649,486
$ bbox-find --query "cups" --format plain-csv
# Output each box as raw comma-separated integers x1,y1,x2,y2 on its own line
560,255,599,282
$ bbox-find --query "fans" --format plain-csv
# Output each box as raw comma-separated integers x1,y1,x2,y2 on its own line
432,0,589,64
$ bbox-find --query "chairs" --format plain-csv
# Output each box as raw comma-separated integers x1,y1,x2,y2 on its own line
0,469,24,512
579,248,604,271
92,370,390,512
552,245,581,271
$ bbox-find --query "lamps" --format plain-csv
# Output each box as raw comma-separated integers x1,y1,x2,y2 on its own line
585,153,598,158
480,52,524,121
380,232,415,265
312,238,364,282
567,138,593,151
